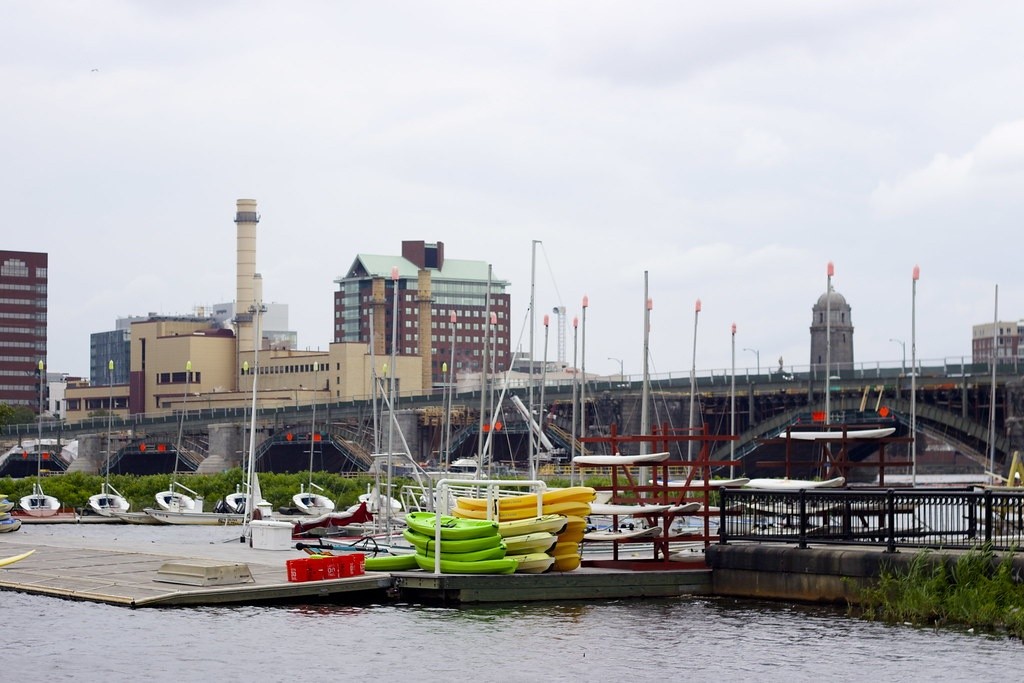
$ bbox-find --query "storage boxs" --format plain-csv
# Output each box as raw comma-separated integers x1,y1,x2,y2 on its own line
284,553,366,582
248,519,295,550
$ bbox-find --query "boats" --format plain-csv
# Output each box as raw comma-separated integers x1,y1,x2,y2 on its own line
309,484,597,576
111,511,159,524
380,463,444,476
144,508,245,526
0,495,21,534
411,458,486,485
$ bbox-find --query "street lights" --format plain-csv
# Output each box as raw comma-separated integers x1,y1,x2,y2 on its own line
606,357,623,384
744,347,759,378
889,339,906,377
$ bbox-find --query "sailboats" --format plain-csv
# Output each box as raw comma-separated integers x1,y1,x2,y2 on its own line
293,360,335,515
357,363,403,515
88,359,130,516
155,360,197,511
226,361,273,519
19,361,62,516
420,364,456,513
